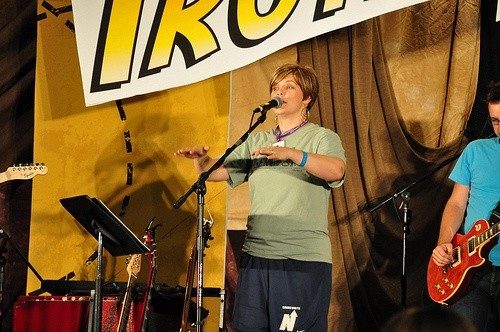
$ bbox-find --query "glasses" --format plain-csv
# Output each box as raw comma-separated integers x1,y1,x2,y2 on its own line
488,114,500,125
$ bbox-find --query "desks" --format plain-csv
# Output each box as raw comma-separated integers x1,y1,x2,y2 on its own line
13,296,179,332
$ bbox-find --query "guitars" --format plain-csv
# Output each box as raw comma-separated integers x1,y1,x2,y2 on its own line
137,224,157,332
116,251,142,332
0,163,48,184
179,219,215,332
428,219,499,305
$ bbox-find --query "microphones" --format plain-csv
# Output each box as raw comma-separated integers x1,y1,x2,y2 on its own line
252,96,282,113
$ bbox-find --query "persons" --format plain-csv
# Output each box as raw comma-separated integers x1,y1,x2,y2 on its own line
173,63,346,332
431,78,500,332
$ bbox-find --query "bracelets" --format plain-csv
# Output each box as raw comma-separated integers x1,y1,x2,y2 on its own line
298,151,308,168
